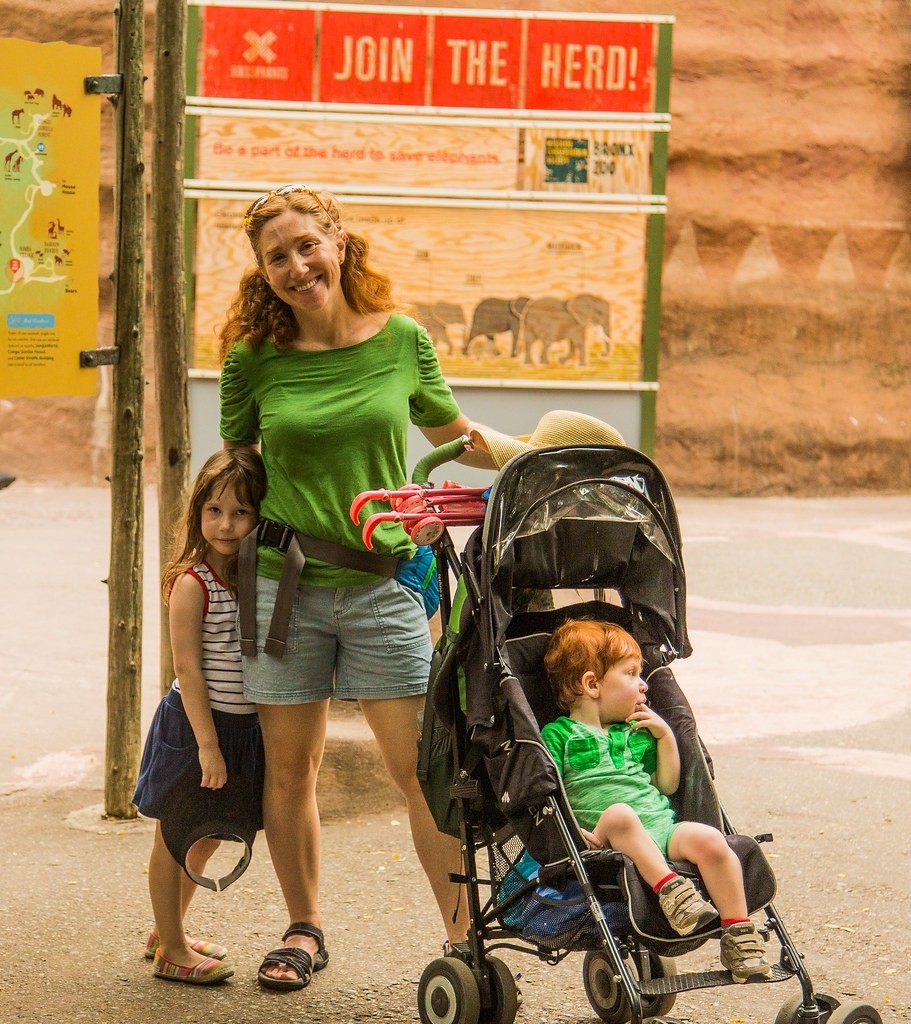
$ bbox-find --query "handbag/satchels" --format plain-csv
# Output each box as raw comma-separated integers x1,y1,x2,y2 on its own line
416,579,503,835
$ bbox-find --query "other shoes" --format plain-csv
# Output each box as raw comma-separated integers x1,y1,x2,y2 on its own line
151,948,233,984
145,931,227,961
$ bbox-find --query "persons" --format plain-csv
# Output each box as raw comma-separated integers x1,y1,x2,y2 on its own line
131,184,531,992
541,618,774,984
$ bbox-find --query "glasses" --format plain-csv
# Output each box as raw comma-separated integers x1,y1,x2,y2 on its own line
251,184,338,232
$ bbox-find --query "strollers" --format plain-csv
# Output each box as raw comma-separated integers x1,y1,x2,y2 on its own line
410,434,883,1023
349,482,490,552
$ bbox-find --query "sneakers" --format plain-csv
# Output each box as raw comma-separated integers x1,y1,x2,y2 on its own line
658,876,718,936
720,923,772,983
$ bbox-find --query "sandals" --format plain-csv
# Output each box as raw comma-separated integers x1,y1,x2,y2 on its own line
259,919,329,988
444,938,522,1007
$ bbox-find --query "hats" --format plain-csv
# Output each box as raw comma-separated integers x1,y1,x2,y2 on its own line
466,408,627,473
163,780,258,893
